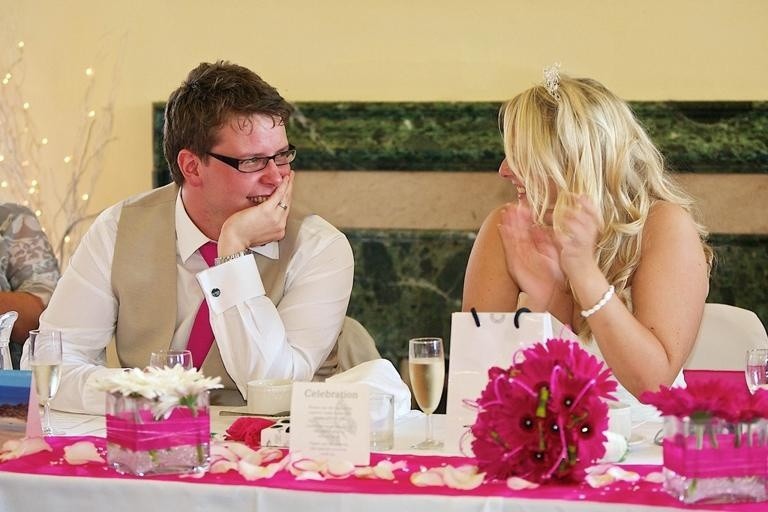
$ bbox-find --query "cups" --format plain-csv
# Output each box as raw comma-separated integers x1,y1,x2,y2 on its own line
606,403,633,443
246,375,293,413
371,394,394,452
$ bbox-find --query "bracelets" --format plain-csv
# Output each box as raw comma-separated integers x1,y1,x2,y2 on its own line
575,282,617,319
214,249,252,267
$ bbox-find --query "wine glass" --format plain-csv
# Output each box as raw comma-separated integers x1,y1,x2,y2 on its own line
147,349,194,372
407,335,447,448
744,347,768,393
27,329,65,436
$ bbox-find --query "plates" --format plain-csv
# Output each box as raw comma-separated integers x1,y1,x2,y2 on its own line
622,435,646,446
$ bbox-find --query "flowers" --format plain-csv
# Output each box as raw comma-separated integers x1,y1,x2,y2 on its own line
456,334,623,487
92,366,227,465
635,378,768,495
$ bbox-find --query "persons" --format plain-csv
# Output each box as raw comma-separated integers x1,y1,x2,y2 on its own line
0,200,64,372
458,58,716,413
16,57,356,420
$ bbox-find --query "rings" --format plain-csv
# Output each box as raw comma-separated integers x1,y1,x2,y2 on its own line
277,200,289,210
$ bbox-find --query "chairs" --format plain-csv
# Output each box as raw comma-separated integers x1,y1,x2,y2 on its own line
314,312,378,381
673,299,765,389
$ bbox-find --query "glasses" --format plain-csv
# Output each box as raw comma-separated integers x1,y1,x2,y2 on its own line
204,142,297,173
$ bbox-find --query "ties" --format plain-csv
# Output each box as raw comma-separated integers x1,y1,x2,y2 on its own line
184,242,225,375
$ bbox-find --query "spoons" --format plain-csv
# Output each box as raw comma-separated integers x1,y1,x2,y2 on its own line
218,407,289,419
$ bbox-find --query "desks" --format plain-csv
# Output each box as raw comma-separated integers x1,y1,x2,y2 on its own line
0,401,767,512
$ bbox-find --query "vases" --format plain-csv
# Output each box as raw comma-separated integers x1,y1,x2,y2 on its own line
102,396,211,478
658,410,767,503
